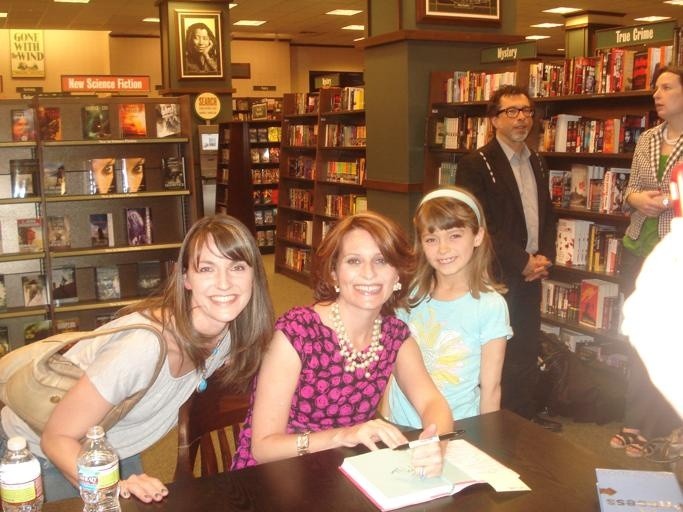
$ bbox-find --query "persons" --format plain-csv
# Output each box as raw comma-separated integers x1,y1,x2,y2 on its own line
455,84,565,432
182,21,218,74
231,212,454,478
380,184,516,430
0,214,272,503
610,66,683,457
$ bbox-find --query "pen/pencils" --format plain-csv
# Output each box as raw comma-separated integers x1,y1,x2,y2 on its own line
391,429,467,451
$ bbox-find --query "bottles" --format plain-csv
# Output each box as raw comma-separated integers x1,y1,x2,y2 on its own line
0,437,44,512
77,426,121,512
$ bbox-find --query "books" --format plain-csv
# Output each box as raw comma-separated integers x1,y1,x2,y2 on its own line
337,436,532,512
439,163,455,186
541,279,618,329
541,322,630,379
214,86,368,277
557,219,622,274
594,465,682,512
434,111,663,153
549,164,631,216
445,42,682,101
1,104,193,351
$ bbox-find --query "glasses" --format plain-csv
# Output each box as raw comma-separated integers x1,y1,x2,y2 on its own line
497,106,535,119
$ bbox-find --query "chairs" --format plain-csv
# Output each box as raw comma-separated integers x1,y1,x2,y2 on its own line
174,370,255,480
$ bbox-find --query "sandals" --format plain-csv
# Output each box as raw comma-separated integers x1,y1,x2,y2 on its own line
610,427,639,449
626,435,649,458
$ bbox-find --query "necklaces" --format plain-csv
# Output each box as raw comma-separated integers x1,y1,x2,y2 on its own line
330,302,383,378
185,338,224,395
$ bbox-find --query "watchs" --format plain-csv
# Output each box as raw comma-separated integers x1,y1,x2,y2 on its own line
297,430,312,456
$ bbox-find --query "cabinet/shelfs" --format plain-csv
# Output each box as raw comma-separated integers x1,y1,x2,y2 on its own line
216,86,365,290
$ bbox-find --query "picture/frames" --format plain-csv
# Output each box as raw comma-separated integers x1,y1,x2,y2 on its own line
173,8,226,82
415,0,502,29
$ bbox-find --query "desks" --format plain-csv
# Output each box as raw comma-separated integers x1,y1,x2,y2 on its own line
43,409,629,512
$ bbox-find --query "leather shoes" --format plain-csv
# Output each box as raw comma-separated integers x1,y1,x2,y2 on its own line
529,414,562,433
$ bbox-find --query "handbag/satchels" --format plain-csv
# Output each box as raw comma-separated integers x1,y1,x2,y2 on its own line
517,328,627,426
641,437,683,484
0,324,168,446
